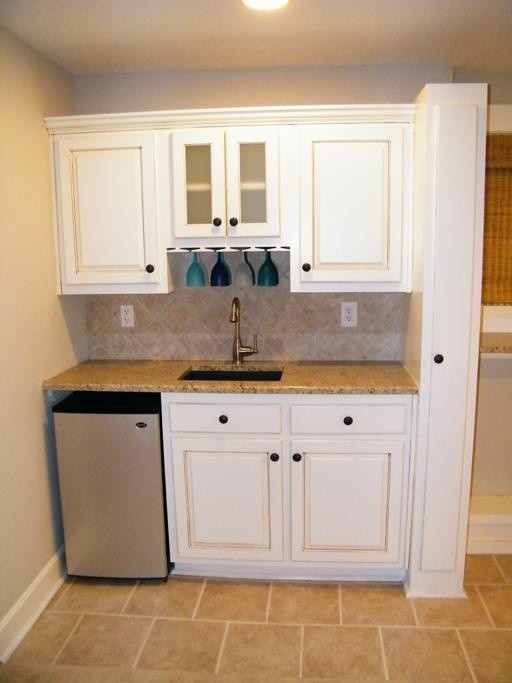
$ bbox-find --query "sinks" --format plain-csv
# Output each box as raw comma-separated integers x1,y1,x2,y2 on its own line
177,365,285,382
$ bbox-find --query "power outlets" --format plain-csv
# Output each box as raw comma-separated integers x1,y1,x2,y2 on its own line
340,301,359,330
119,304,135,329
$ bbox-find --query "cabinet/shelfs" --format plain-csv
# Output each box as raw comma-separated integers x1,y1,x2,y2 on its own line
161,393,414,583
51,119,419,294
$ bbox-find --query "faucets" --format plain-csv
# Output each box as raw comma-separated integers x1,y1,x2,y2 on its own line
228,296,258,365
